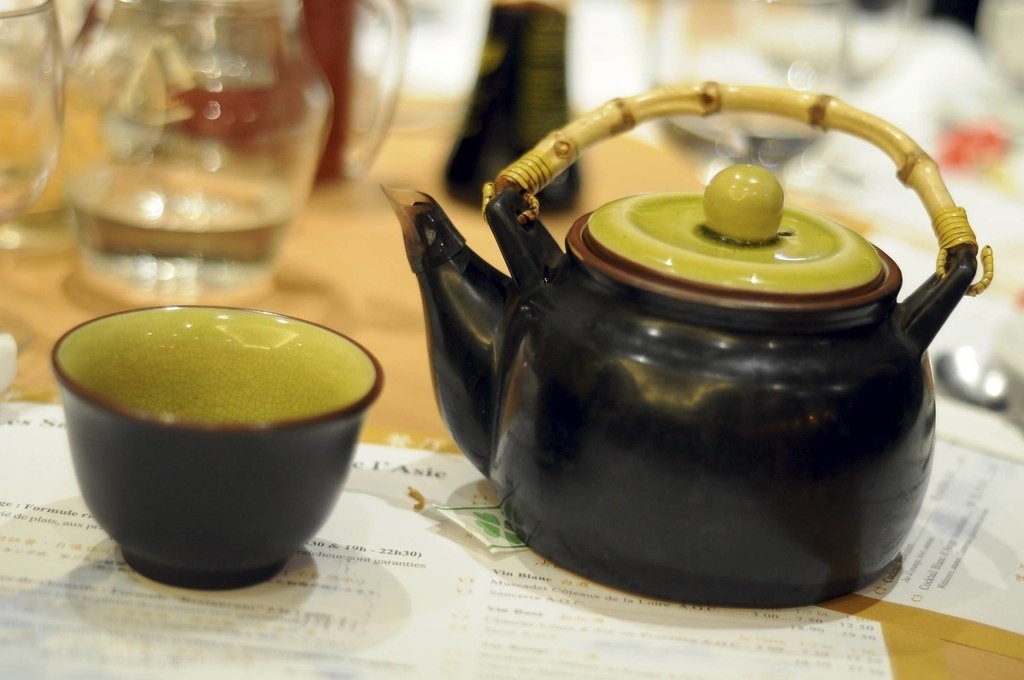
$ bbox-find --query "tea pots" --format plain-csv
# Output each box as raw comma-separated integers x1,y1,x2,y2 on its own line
378,82,996,608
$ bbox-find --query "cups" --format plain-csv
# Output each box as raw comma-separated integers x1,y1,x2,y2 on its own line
53,304,384,588
650,0,852,189
0,0,61,227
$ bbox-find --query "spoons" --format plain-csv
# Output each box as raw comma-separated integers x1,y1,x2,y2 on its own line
934,344,1024,436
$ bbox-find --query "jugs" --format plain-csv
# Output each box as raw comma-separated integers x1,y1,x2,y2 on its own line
63,0,408,313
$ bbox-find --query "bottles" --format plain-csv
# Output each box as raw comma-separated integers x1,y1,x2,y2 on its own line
442,0,581,214
